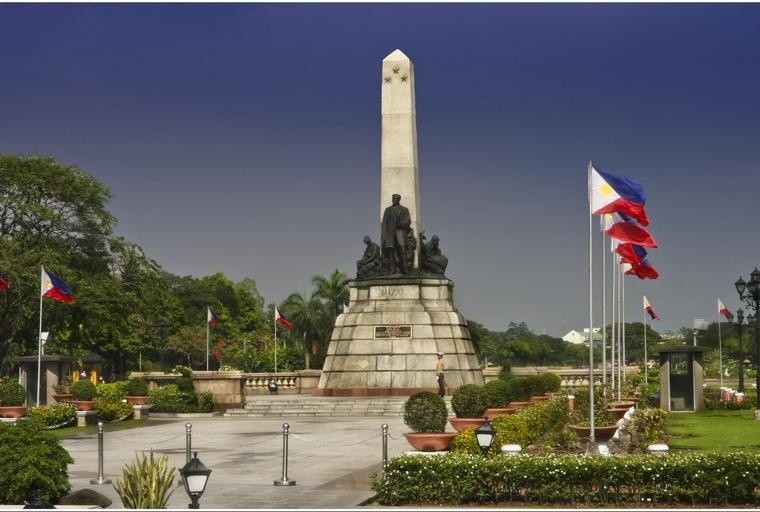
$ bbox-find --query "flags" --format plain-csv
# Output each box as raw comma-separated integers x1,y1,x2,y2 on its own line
275,307,281,320
42,267,72,297
208,308,213,321
719,301,734,323
644,296,659,321
592,167,659,280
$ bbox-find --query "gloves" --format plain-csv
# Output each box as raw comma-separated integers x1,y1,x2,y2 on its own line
436,376,440,380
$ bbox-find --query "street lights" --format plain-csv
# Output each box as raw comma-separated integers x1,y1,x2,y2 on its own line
733,265,760,423
726,307,756,393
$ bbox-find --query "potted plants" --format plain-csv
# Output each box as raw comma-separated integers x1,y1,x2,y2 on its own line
567,378,642,443
447,382,490,434
67,378,99,411
505,372,562,409
50,382,74,403
402,391,458,451
481,379,518,423
0,377,29,418
122,376,150,405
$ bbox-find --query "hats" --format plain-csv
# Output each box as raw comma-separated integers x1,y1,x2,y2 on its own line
437,352,444,356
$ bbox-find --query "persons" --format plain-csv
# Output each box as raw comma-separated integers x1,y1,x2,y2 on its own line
382,194,412,273
436,352,445,397
357,236,380,277
421,236,448,274
